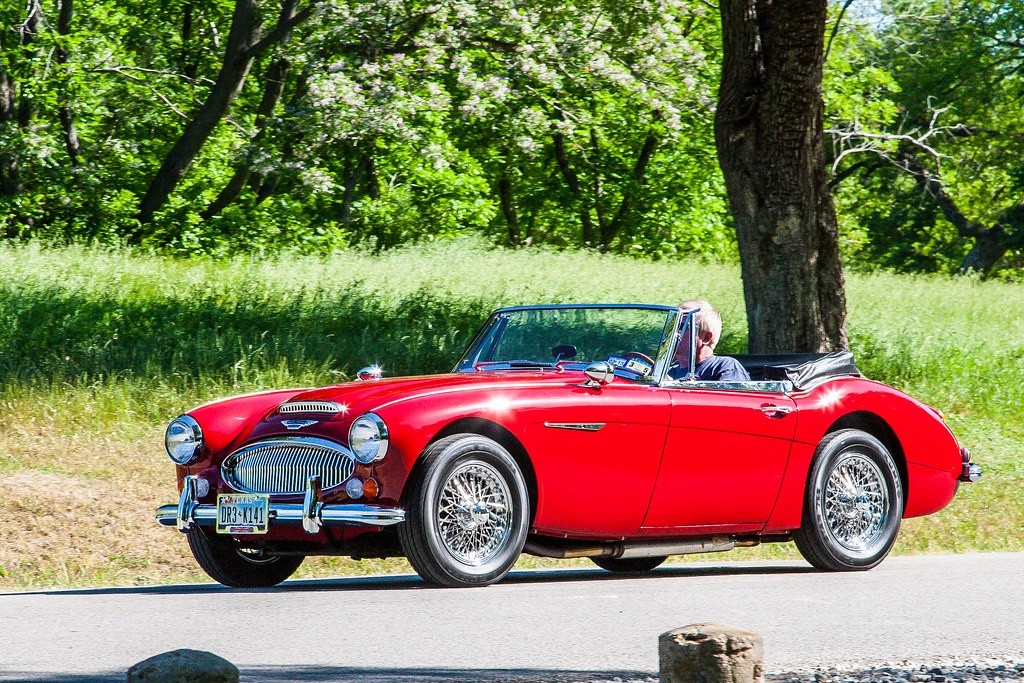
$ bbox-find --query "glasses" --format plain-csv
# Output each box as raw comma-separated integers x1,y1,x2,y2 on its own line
678,321,698,331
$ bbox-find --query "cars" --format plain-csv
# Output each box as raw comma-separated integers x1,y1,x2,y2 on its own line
156,303,985,588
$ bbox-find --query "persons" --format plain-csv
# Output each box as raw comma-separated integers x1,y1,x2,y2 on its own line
667,300,751,381
